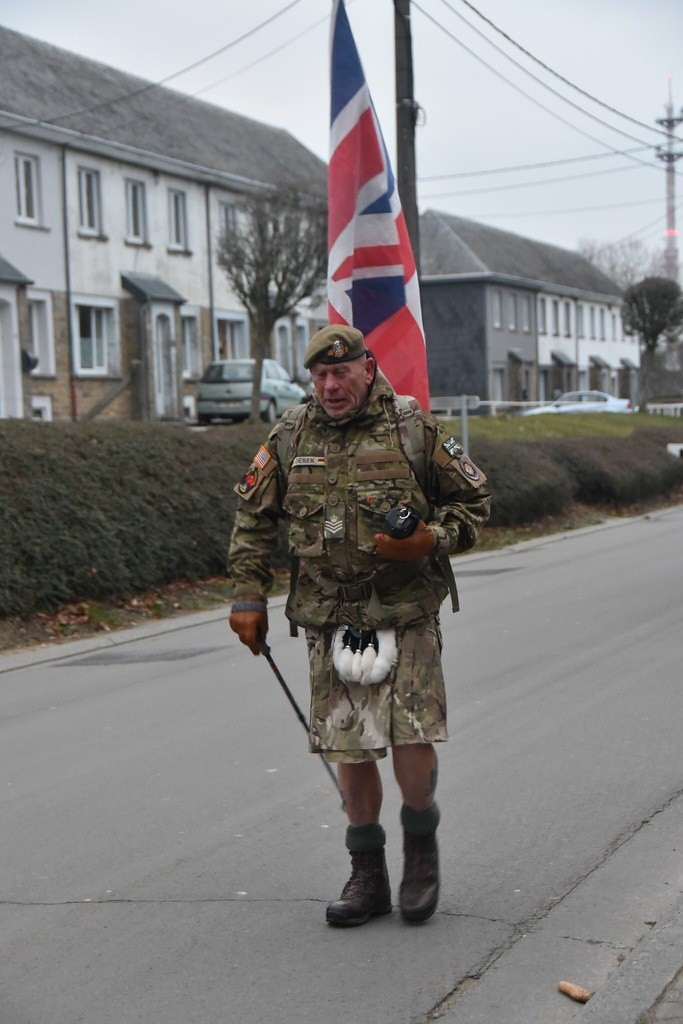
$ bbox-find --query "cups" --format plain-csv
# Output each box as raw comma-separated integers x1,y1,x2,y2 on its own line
383,507,419,539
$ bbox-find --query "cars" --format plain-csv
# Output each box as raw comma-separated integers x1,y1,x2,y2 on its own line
196,359,307,423
519,390,640,415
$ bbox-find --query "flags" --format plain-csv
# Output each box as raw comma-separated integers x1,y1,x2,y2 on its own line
327,0,432,415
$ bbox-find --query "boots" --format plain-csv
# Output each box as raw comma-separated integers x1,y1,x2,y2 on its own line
399,829,441,921
327,848,393,925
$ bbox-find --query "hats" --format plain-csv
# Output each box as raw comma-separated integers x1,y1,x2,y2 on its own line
302,323,367,369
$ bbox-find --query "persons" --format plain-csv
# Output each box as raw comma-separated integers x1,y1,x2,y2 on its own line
228,327,488,927
